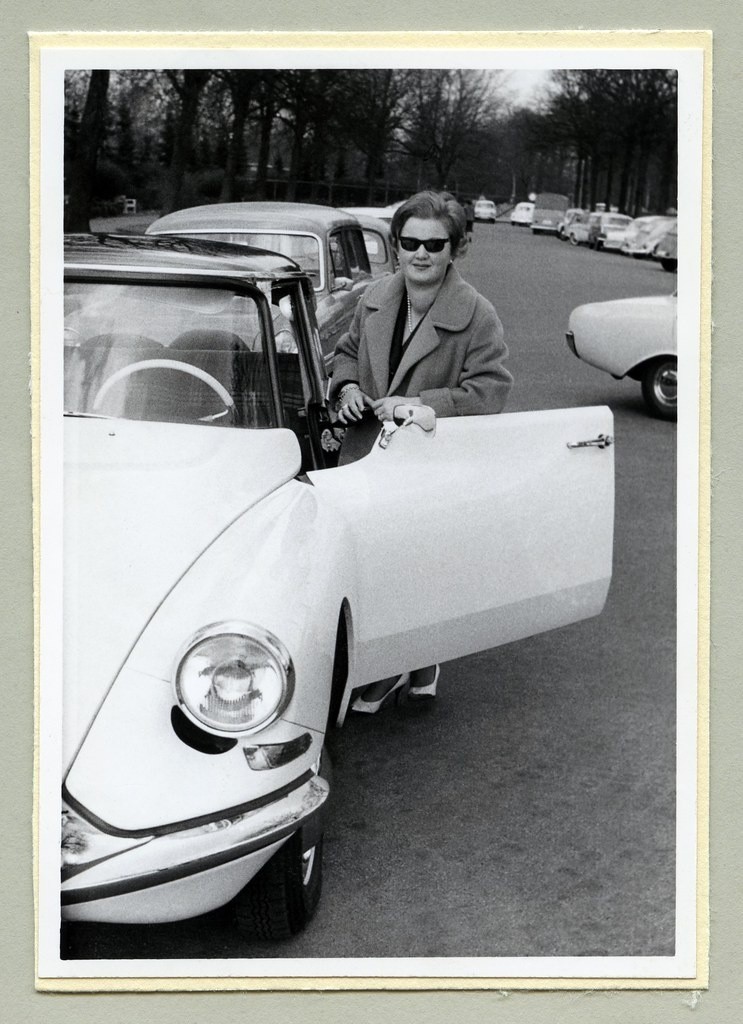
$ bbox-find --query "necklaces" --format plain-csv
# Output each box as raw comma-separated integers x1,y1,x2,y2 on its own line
407,291,418,333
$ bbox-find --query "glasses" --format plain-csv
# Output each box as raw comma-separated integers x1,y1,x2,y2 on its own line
399,236,449,253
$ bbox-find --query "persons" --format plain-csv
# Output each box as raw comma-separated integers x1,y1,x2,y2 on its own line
463,199,474,242
330,193,512,716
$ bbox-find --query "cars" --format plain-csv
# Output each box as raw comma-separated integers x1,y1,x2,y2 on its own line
530,192,678,273
474,199,496,224
62,233,617,940
567,287,678,422
346,212,397,280
140,201,373,375
510,202,534,227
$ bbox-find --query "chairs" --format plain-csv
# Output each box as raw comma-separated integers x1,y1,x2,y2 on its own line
74,329,269,430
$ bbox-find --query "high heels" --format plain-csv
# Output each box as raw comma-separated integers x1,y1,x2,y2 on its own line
408,664,440,701
350,672,408,714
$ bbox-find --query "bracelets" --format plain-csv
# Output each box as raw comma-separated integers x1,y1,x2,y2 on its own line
337,386,360,400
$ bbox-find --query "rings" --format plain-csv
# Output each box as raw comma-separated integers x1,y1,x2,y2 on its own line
380,414,385,421
